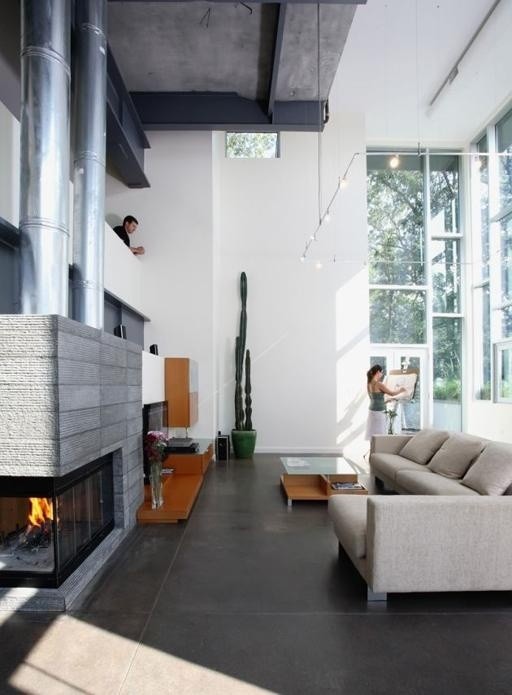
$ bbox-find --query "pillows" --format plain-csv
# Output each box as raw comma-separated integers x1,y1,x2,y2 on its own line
426,432,488,480
398,429,450,466
460,441,511,494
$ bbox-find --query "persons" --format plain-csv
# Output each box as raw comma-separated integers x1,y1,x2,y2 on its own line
113,216,144,255
365,365,407,441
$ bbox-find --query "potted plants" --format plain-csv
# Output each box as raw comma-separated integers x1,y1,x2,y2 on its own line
231,271,258,458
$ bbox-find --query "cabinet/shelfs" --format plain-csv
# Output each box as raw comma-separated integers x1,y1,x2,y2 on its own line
165,357,199,427
136,438,213,524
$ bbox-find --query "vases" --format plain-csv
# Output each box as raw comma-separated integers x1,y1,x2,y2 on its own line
151,462,163,510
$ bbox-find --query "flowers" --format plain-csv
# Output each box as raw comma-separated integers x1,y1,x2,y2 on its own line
148,430,168,500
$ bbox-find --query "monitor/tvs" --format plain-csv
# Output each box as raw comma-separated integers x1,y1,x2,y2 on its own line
143,400,168,485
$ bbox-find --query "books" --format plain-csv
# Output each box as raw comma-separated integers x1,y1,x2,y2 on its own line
332,482,363,489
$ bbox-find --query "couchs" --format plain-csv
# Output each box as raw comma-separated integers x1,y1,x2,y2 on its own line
327,435,512,602
